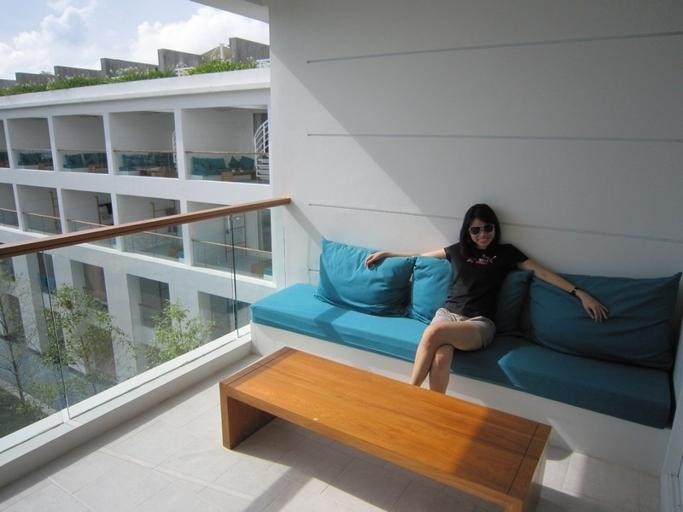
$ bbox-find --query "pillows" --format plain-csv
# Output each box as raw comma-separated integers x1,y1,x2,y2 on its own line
238,155,255,170
526,271,683,369
408,255,534,333
315,236,416,316
228,155,239,169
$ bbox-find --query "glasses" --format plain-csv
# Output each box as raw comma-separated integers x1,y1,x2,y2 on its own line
468,225,494,235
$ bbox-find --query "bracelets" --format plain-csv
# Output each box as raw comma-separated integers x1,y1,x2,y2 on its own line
569,286,579,296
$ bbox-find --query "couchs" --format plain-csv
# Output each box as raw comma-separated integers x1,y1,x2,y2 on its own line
119,153,173,175
191,156,231,180
249,281,675,476
64,152,108,173
18,153,52,170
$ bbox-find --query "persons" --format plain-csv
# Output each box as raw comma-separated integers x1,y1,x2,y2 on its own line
364,203,608,393
